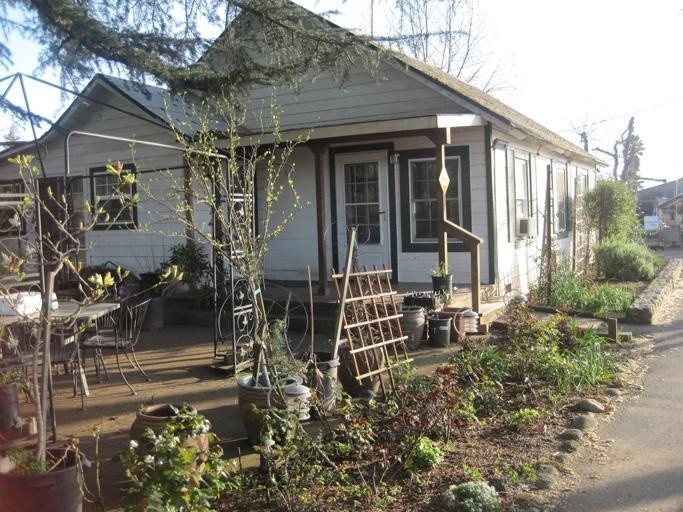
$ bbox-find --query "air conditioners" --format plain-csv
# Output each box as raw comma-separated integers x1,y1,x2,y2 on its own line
516,217,535,237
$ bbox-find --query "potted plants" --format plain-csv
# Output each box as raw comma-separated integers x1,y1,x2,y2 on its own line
330,274,386,399
0,145,147,512
113,81,304,445
430,260,453,298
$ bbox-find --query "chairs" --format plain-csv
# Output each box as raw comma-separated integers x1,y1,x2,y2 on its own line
1,292,151,403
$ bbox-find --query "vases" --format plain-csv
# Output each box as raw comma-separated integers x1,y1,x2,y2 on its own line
289,350,340,419
132,403,208,483
126,274,162,297
389,291,473,350
